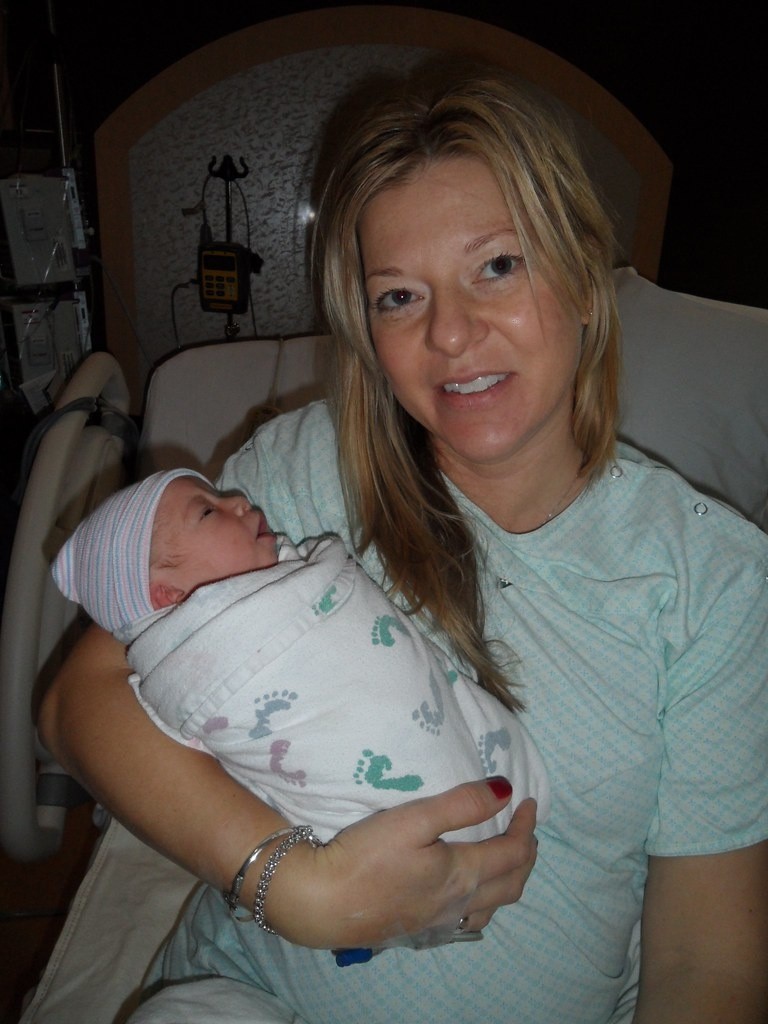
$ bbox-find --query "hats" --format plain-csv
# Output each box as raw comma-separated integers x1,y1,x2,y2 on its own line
50,467,215,631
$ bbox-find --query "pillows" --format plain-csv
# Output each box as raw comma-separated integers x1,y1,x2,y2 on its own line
594,267,768,533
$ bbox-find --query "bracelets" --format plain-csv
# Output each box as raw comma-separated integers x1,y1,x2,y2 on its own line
222,823,325,935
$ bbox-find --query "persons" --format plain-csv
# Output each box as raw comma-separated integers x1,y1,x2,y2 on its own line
113,81,768,1024
48,468,549,845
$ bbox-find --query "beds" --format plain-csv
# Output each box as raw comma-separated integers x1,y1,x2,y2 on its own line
0,333,640,1024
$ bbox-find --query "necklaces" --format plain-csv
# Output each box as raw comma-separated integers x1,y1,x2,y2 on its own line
541,471,577,526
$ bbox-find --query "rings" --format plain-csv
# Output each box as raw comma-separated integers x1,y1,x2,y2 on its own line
455,917,468,935
